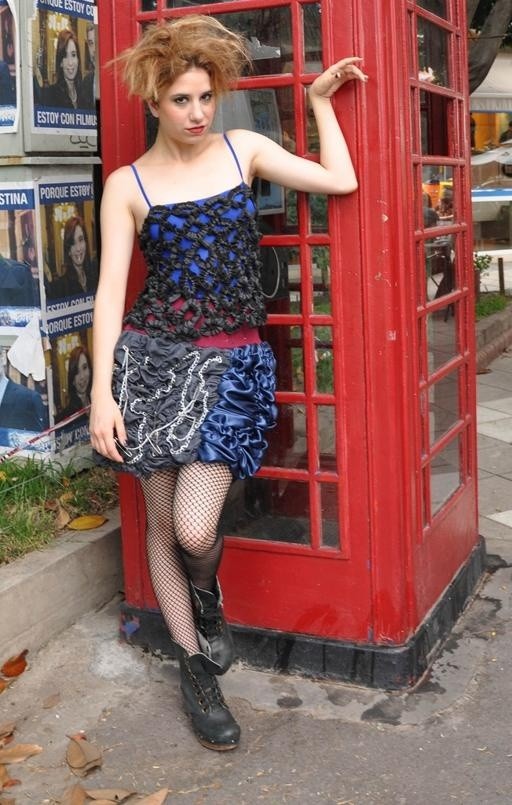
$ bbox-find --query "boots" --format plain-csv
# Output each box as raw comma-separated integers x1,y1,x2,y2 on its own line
188,576,235,677
167,632,240,752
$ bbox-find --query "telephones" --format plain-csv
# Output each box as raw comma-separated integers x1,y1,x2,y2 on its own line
206,88,286,215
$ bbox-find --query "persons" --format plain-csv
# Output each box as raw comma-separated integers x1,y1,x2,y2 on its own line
56,346,92,424
88,13,369,752
41,30,94,110
51,216,99,298
0,345,49,433
